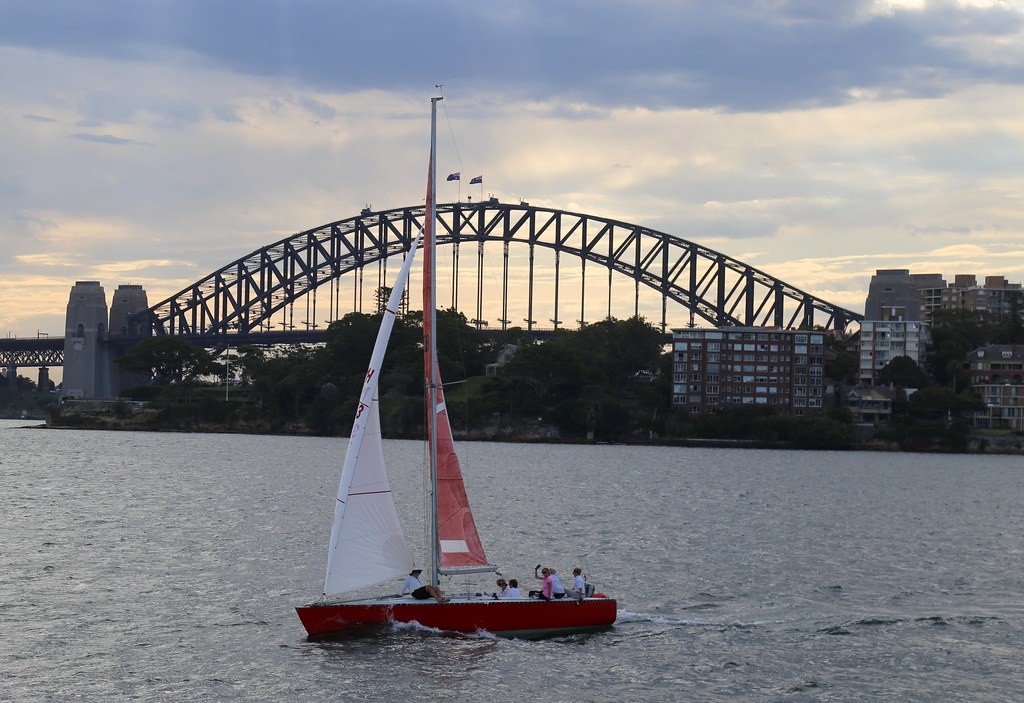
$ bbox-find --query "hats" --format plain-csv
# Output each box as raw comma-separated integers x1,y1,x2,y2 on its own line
409,570,422,576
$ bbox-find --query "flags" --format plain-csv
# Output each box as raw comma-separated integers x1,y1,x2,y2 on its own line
447,173,460,181
470,176,482,184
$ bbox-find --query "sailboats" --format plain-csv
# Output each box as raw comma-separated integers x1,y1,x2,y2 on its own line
295,98,617,638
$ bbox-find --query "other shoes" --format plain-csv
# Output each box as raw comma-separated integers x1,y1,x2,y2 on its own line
439,597,451,604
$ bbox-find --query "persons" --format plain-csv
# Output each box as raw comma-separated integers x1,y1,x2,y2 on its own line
549,569,565,598
393,570,450,602
505,579,520,597
496,579,508,597
564,568,585,599
529,568,553,600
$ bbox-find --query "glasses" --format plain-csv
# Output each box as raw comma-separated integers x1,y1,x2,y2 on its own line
500,583,505,585
542,571,547,574
